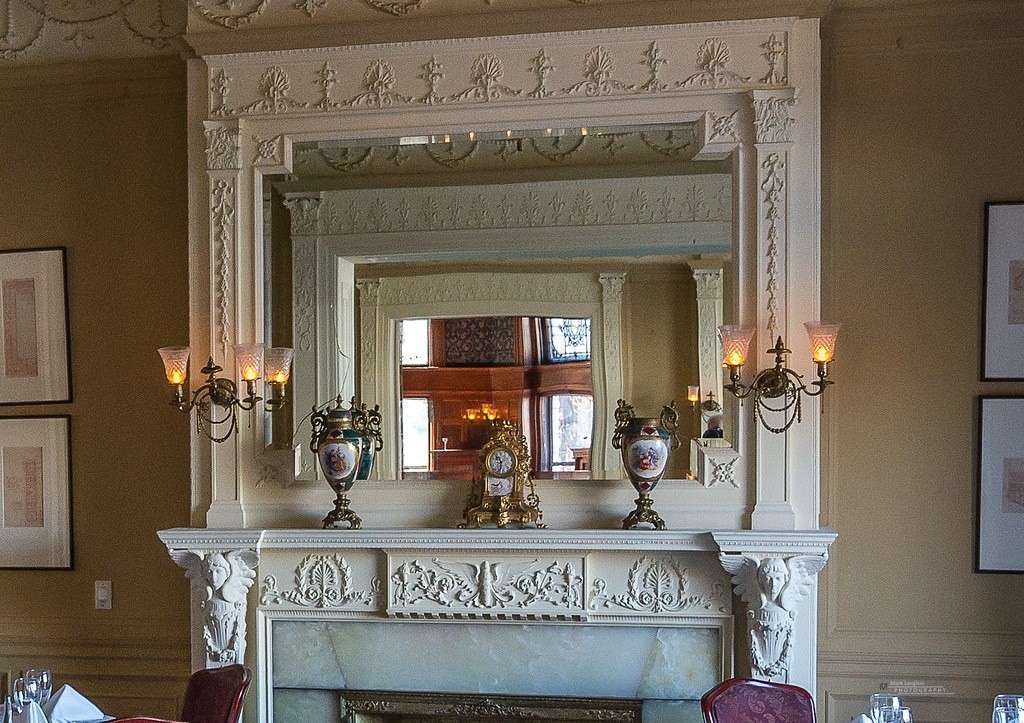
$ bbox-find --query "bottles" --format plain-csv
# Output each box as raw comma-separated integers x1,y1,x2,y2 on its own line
1,695,14,723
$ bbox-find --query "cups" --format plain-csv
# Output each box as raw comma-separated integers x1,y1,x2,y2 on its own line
14,668,53,714
991,694,1024,723
869,693,913,723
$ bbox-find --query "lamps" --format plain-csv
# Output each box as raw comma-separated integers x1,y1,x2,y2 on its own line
718,320,843,434
158,344,295,442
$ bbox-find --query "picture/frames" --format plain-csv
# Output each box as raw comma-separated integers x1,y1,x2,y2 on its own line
973,393,1024,576
980,199,1024,382
0,414,75,570
0,246,73,407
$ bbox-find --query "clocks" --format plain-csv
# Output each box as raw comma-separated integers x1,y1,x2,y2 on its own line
457,417,549,531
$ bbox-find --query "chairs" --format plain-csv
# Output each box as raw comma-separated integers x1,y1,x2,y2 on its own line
699,677,818,723
102,664,252,723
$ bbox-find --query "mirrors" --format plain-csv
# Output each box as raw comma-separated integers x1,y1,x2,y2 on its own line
187,14,821,536
